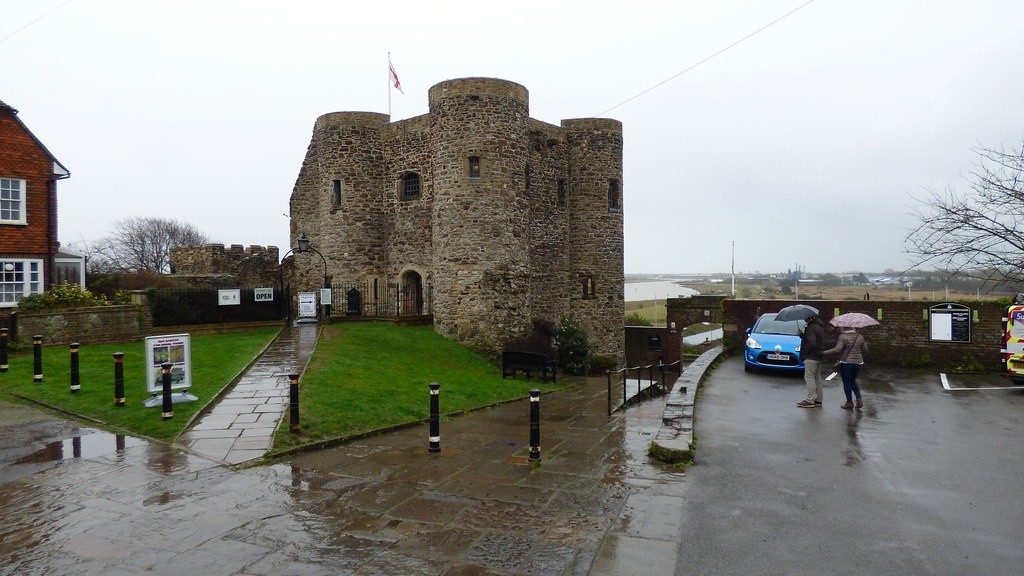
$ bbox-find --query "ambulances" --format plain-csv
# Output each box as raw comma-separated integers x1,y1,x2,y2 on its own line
999,293,1024,386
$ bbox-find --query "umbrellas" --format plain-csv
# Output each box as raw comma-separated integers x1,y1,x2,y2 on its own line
829,312,880,333
775,303,820,329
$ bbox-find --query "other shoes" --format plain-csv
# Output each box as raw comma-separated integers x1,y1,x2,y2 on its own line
814,401,822,407
797,400,814,408
855,398,863,408
841,402,853,409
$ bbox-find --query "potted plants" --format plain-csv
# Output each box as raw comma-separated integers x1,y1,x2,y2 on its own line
553,314,620,376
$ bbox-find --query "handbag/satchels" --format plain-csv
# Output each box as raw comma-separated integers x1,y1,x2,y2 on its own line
833,359,845,372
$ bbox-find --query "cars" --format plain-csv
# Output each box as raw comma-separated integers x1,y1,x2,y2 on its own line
743,312,809,377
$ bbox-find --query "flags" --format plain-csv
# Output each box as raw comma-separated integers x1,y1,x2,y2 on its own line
390,57,404,95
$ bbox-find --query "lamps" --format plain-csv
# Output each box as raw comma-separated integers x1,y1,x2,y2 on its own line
161,364,173,421
528,388,542,462
297,232,309,253
113,352,125,407
0,327,9,372
70,342,81,394
5,262,14,271
428,382,441,453
33,334,44,382
289,373,301,434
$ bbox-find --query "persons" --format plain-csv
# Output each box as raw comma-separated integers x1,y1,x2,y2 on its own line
821,327,868,410
797,316,826,408
